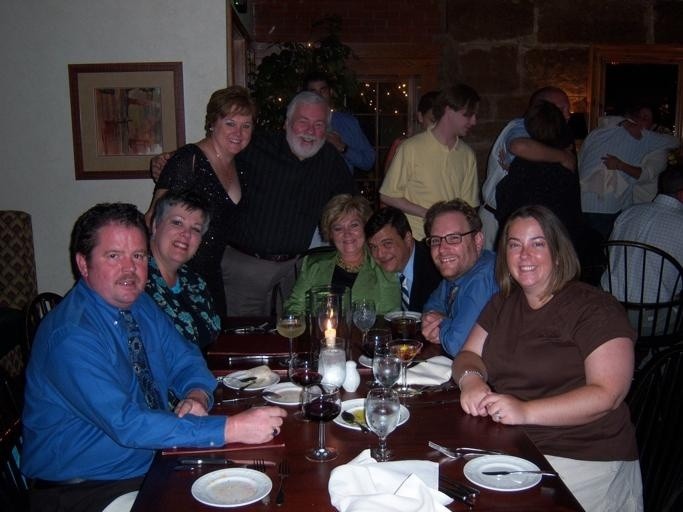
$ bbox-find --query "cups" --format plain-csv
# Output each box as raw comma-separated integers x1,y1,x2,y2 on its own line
307,285,353,372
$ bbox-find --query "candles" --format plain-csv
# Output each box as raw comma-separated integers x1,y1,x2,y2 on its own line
323,322,338,344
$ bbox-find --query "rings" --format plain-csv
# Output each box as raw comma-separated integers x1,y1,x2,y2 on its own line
495,412,502,419
273,426,278,436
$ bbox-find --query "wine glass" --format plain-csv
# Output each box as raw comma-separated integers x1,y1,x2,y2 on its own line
365,388,400,463
366,328,392,385
289,351,325,422
300,384,341,462
385,338,424,397
372,348,401,416
316,336,347,404
317,308,339,333
354,298,376,343
277,310,306,365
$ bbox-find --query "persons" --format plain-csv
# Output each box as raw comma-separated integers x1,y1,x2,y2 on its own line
451,204,643,510
18,204,287,510
142,71,683,375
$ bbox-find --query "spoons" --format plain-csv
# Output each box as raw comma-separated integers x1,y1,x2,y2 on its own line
221,391,281,403
340,410,369,434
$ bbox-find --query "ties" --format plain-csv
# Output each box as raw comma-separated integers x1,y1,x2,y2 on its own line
120,312,165,411
398,273,411,312
445,284,460,321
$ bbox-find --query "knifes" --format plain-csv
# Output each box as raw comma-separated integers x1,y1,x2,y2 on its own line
484,471,558,476
181,459,277,467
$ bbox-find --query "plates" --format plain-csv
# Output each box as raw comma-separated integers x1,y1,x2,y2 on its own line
332,396,410,431
261,381,317,405
464,454,543,493
190,468,274,509
222,369,281,391
384,310,422,323
358,355,400,368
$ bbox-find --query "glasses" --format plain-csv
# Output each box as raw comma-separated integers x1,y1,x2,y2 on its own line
425,227,478,247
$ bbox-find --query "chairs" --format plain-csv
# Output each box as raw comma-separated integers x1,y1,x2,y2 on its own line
21,292,65,371
0,365,34,510
624,341,682,511
579,240,683,420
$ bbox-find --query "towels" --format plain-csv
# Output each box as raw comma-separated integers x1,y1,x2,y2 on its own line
328,449,455,512
396,355,453,386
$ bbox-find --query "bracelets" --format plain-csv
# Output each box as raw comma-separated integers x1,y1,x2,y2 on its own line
458,370,483,390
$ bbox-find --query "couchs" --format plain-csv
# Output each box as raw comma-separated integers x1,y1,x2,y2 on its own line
0,209,37,387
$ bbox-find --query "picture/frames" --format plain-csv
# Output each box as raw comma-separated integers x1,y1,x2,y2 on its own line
67,60,186,181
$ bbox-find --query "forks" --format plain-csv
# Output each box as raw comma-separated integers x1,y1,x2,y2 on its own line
274,457,290,506
252,457,265,471
429,440,501,459
